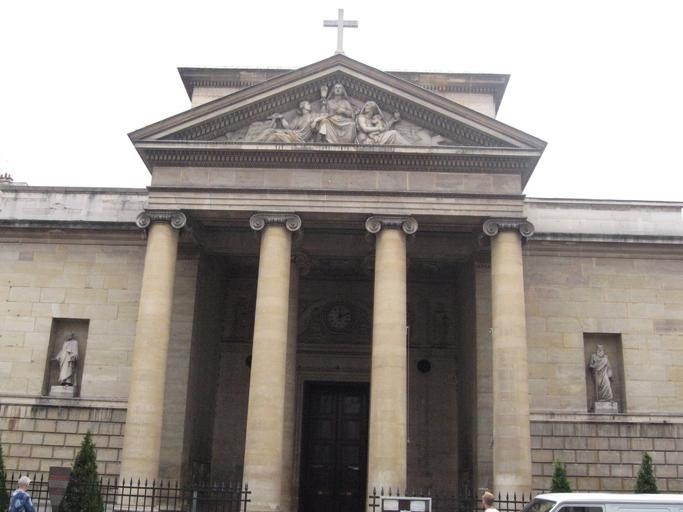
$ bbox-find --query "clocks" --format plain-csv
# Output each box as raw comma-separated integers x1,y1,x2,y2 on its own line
322,297,355,333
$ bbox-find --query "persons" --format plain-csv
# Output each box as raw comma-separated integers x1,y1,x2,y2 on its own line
355,99,410,144
480,490,499,512
46,329,81,386
262,99,334,143
314,82,358,145
7,476,37,511
585,341,618,402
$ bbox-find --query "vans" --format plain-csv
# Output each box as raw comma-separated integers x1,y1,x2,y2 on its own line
517,491,683,511
